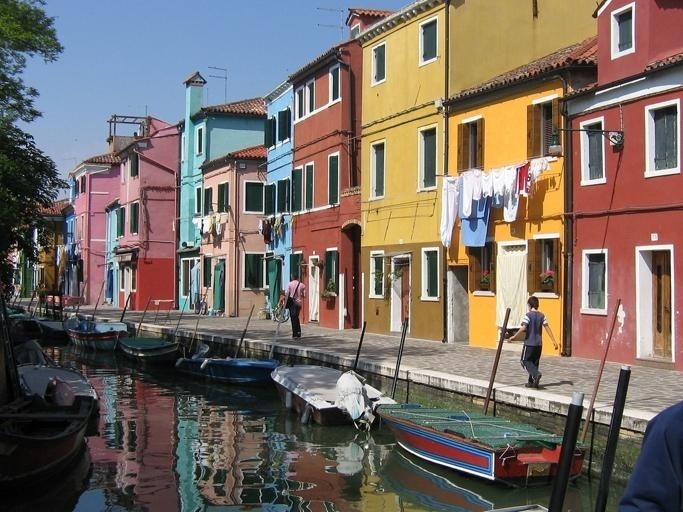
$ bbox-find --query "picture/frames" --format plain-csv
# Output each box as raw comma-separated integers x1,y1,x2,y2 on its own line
540,282,553,291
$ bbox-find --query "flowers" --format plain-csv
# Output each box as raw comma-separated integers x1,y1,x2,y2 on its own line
480,271,488,283
539,269,553,282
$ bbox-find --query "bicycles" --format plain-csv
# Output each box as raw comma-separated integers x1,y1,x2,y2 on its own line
195,293,208,315
271,290,291,323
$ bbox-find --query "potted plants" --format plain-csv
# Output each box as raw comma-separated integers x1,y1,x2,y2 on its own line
324,278,337,298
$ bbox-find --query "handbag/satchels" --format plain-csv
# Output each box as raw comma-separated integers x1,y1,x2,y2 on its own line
285,297,293,308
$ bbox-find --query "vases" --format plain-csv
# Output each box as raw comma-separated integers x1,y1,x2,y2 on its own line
480,281,488,291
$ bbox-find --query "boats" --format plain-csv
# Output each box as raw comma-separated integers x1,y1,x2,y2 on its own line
117,334,188,375
0,303,99,512
376,401,587,490
65,315,129,352
175,355,280,388
271,363,398,427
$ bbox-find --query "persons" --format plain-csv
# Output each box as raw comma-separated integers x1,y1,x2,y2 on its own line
284,274,305,338
34,279,47,308
507,296,559,389
619,400,682,510
273,290,286,321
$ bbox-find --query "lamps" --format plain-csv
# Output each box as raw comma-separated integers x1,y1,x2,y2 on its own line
548,126,623,158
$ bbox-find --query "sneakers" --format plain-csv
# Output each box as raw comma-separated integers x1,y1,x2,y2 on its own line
525,383,539,387
533,374,541,384
